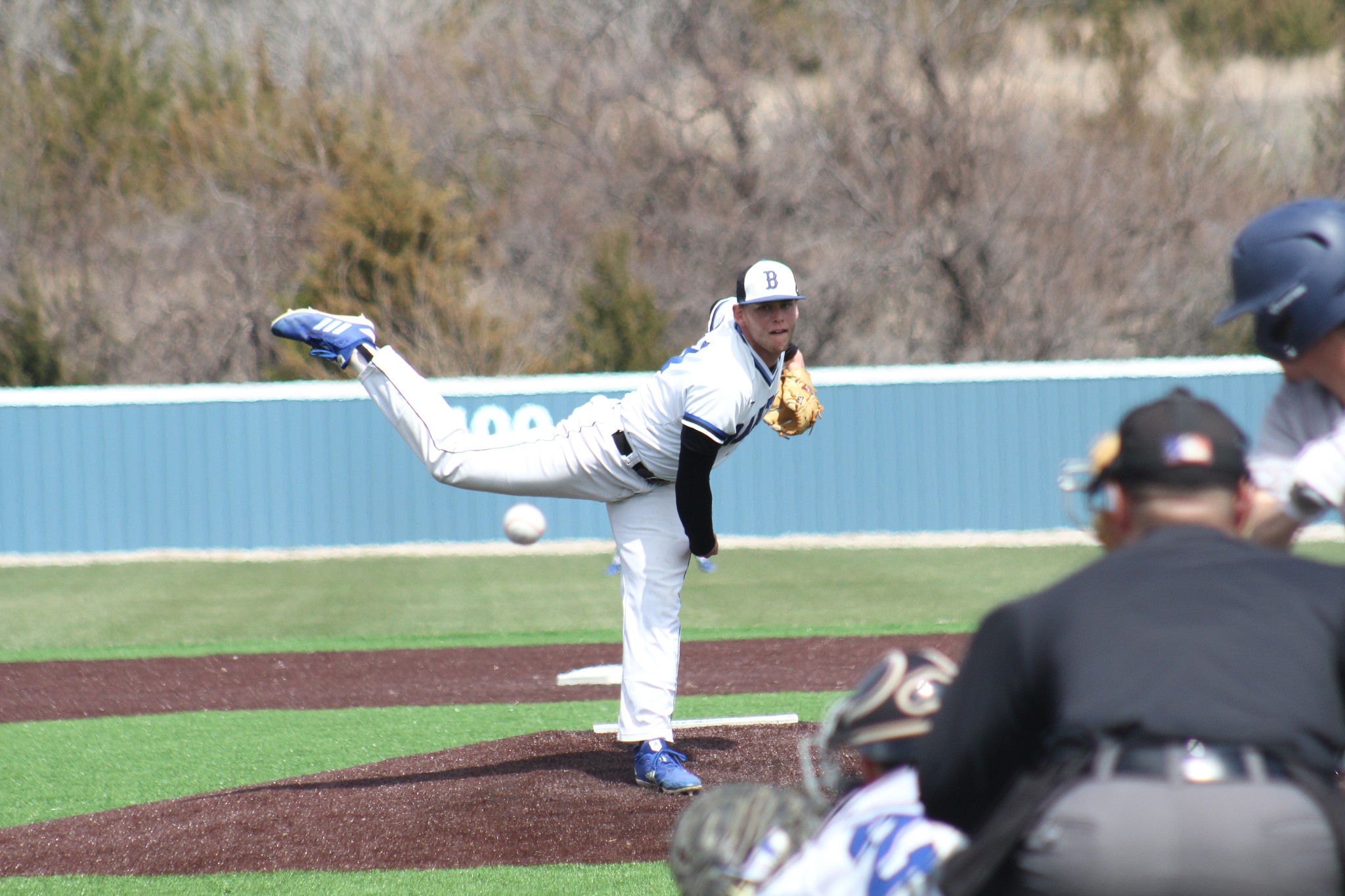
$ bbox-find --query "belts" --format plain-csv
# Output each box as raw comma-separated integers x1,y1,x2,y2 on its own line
611,430,669,488
1114,739,1284,784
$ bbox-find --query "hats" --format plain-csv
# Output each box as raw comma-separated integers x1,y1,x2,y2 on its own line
1082,386,1251,491
736,258,805,306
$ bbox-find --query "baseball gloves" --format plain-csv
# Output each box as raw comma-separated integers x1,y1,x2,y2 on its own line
762,367,824,441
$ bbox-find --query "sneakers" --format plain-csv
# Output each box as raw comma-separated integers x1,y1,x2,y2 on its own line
269,306,377,369
634,737,701,792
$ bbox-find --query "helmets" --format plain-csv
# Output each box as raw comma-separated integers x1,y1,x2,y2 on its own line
1213,201,1345,360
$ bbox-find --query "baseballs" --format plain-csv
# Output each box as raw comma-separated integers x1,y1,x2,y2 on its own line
502,503,548,545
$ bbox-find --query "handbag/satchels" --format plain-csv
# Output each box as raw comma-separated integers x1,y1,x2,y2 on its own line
934,750,1094,896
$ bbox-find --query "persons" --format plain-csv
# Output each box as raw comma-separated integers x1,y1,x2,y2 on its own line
917,386,1345,896
671,647,969,896
1211,199,1345,551
269,260,824,792
